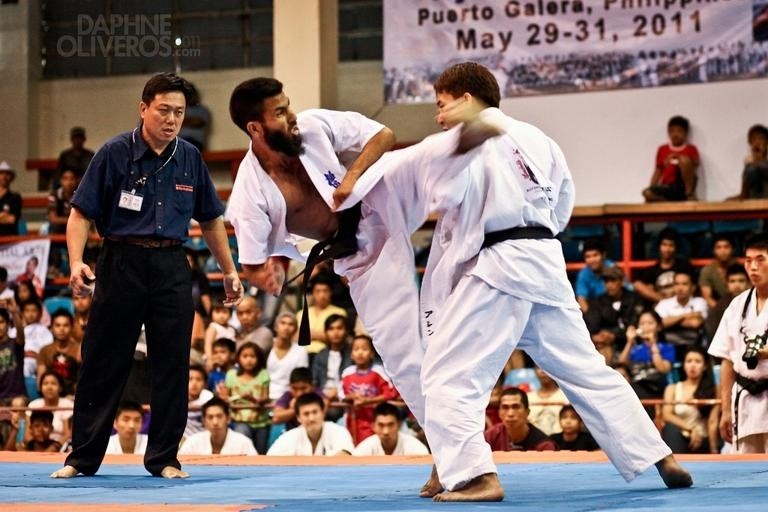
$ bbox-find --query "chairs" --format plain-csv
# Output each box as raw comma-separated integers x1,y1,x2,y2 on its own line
0,215,768,449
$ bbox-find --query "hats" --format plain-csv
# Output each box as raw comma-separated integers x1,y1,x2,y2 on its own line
0,161,16,174
599,266,624,280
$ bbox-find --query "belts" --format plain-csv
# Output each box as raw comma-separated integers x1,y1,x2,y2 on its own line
108,235,182,248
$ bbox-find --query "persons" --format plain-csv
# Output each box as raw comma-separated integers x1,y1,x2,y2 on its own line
46,167,94,279
50,73,244,478
224,77,507,498
56,127,95,179
0,160,22,236
0,267,152,454
176,85,210,153
724,124,768,200
642,116,700,201
419,62,692,501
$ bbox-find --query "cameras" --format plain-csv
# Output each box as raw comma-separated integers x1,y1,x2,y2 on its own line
741,336,764,370
